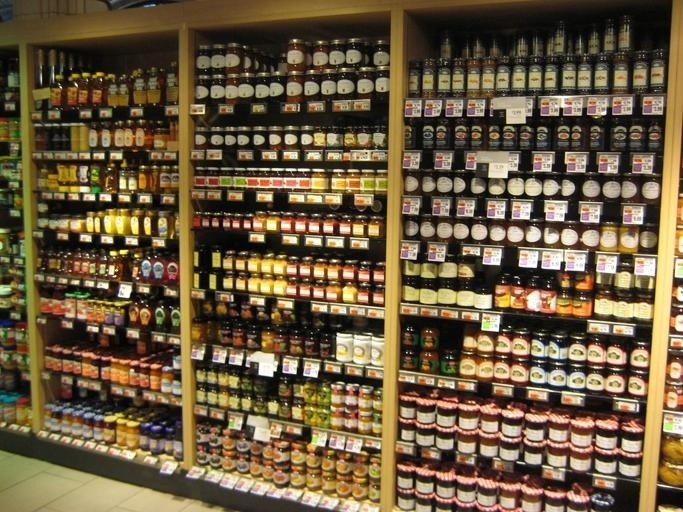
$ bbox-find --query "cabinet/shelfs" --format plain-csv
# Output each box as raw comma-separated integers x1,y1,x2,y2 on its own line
0,0,683,512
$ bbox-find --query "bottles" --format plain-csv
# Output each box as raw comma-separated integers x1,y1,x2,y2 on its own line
0,49,178,115
